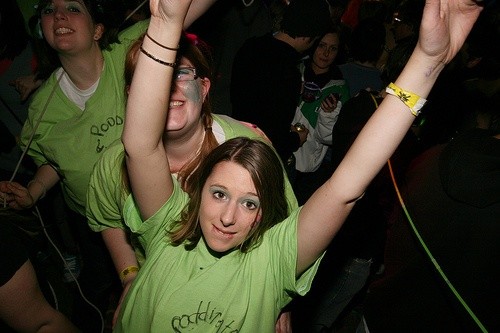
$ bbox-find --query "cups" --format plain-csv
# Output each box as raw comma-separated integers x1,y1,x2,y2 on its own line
301,81,322,103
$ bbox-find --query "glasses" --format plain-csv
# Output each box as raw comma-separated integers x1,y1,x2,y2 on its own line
173,67,204,82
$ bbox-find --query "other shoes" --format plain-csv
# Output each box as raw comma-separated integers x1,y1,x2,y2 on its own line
344,258,385,277
62,255,81,282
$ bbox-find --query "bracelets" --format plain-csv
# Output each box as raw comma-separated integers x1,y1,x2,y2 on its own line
386,83,427,117
27,180,46,200
146,33,179,51
120,266,140,283
140,44,176,68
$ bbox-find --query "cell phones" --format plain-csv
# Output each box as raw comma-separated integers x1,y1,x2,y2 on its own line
323,93,340,108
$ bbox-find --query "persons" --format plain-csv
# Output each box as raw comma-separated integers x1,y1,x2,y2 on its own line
234,11,318,183
116,0,482,332
0,218,82,333
2,0,212,211
223,2,497,131
85,31,299,333
292,30,347,172
